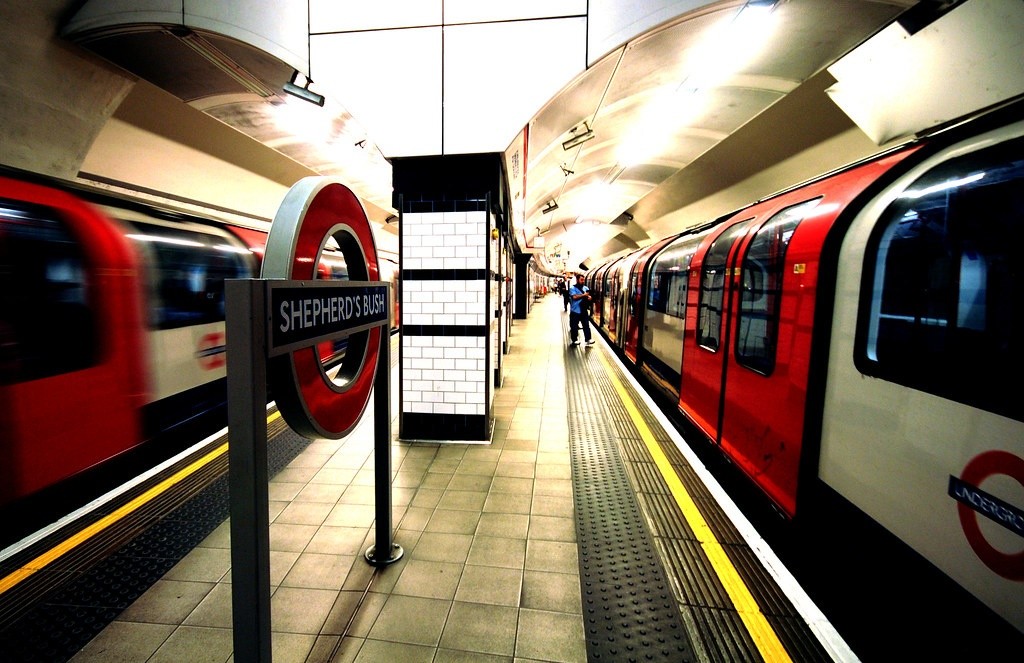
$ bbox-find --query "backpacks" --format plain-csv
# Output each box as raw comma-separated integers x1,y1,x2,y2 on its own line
579,290,595,310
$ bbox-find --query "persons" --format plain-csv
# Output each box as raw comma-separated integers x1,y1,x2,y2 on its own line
564,289,571,311
554,276,566,295
569,274,595,344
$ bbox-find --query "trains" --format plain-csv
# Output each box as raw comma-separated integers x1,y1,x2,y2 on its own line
580,89,1024,632
1,13,402,518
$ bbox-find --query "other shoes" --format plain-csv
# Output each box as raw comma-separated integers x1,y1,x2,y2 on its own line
573,339,595,346
